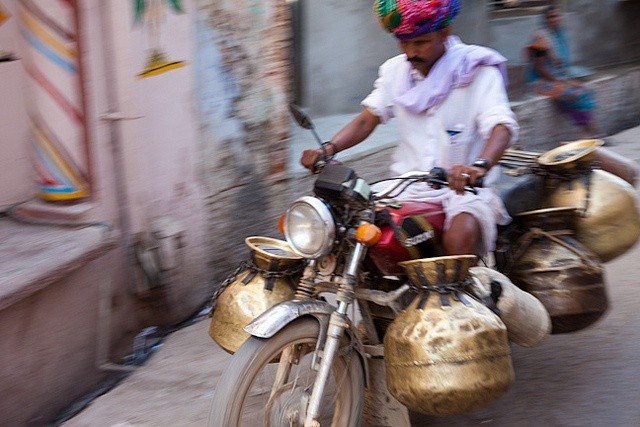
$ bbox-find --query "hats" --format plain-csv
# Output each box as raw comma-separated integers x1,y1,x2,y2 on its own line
373,0,463,38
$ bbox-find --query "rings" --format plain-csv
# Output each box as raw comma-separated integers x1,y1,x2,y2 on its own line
460,172,471,178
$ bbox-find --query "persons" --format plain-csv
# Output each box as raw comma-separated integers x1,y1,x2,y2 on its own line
518,5,606,147
300,0,520,269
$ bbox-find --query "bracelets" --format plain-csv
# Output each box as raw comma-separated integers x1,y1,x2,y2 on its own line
471,157,496,170
321,141,337,155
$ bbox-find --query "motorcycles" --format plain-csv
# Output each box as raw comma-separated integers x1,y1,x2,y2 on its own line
209,104,549,427
